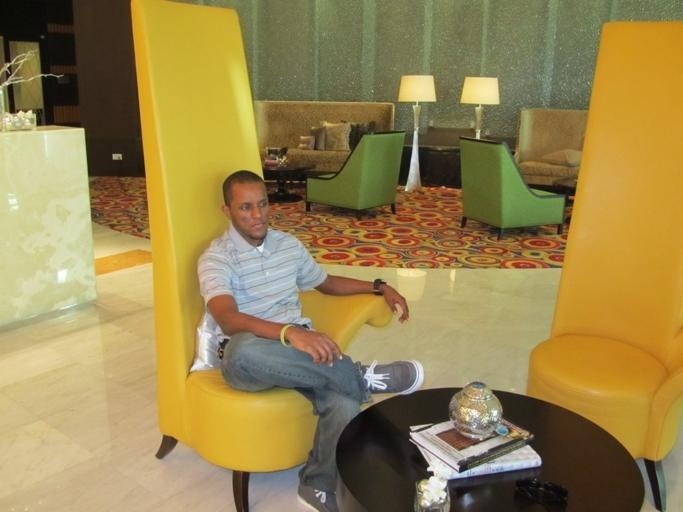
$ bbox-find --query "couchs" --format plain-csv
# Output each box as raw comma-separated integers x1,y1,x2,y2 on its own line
513,108,590,187
459,136,566,241
252,99,395,175
305,128,407,221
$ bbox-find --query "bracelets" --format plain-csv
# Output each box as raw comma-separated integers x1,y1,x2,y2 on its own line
280,323,294,346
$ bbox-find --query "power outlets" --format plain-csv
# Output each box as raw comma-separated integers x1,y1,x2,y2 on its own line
112,153,123,160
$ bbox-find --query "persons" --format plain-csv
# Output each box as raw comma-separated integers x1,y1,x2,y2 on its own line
195,167,423,511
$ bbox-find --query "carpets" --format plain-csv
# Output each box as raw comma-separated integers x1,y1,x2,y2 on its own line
88,175,575,269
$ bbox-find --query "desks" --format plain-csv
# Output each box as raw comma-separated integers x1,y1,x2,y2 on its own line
337,385,646,512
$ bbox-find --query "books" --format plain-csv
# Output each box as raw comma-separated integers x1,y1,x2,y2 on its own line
406,417,542,480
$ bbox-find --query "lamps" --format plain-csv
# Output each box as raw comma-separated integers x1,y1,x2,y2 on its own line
397,75,437,192
460,77,501,139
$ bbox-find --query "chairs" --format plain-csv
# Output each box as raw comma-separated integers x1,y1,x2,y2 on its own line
528,19,682,512
130,0,394,511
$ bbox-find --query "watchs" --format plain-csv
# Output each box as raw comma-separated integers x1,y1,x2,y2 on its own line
373,278,384,295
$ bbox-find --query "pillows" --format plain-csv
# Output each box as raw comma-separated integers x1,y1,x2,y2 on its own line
299,118,377,151
541,149,583,167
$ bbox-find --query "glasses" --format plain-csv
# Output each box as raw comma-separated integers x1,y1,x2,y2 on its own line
516,477,567,505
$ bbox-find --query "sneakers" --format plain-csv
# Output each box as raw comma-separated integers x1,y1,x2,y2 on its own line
297,482,337,512
362,360,424,394
